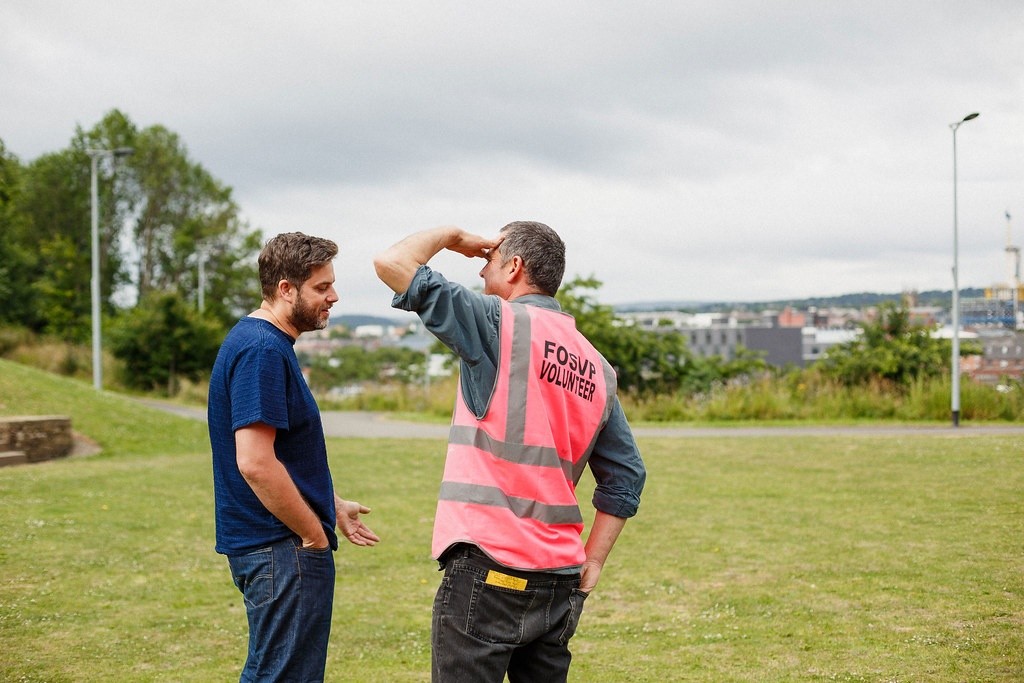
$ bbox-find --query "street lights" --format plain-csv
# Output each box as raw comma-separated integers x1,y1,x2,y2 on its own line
88,145,135,392
947,111,981,428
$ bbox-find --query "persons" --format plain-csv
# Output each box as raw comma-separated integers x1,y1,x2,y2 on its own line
374,221,647,683
209,232,381,683
